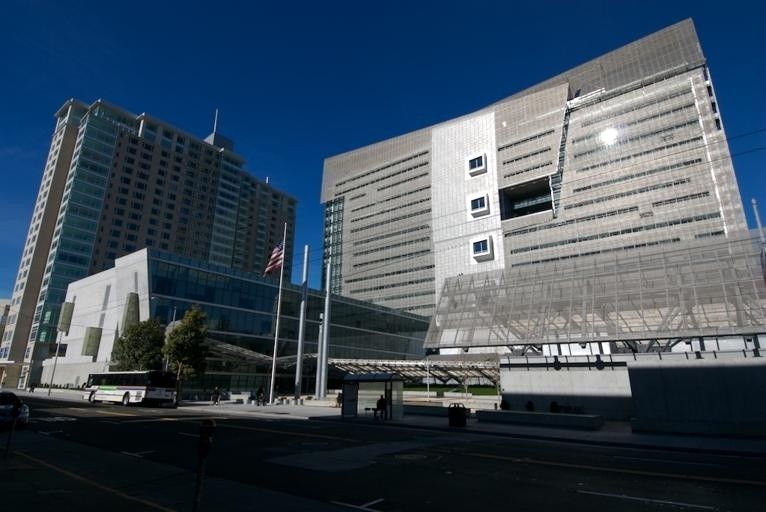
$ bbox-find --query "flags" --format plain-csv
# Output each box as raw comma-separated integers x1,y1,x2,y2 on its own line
263,239,285,277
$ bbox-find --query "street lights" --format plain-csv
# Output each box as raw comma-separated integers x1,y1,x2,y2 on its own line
44,323,62,396
151,295,177,372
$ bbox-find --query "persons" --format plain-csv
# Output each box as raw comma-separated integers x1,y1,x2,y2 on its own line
210,386,226,404
334,392,342,407
29,382,37,393
256,393,267,406
374,395,387,418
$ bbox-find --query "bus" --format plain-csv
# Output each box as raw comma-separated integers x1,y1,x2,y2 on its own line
82,370,177,407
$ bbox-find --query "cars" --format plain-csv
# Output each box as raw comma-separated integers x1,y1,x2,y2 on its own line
0,390,29,424
